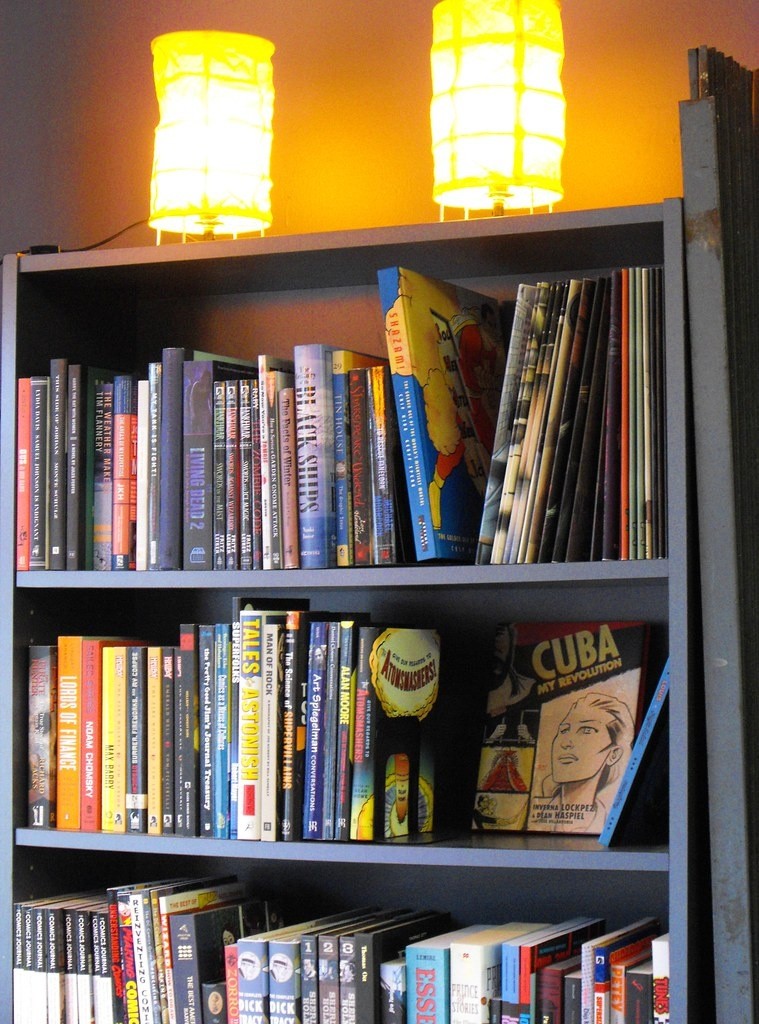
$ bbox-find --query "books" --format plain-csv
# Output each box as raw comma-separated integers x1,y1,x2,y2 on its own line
14,263,669,570
12,877,670,1024
26,595,678,846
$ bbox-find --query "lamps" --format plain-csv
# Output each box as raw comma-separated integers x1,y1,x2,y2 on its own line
146,29,276,245
427,0,570,225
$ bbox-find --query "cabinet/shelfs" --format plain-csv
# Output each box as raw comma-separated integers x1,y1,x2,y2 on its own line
0,198,692,1024
